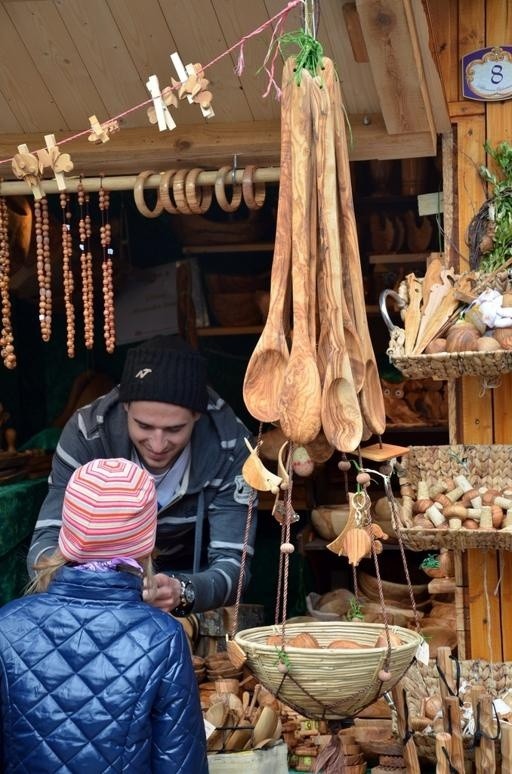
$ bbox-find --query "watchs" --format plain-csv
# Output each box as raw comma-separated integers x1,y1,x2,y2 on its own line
170,575,195,611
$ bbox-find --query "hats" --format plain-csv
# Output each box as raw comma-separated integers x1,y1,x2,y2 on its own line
58,457,158,564
120,334,208,413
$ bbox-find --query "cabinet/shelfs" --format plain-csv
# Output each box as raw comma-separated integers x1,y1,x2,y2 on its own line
386,348,511,552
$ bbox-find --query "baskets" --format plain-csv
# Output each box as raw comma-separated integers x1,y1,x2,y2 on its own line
381,271,511,379
235,621,424,721
386,444,512,552
391,659,511,766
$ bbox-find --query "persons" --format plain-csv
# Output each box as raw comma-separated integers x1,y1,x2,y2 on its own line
0,458,208,773
29,331,257,618
185,168,212,215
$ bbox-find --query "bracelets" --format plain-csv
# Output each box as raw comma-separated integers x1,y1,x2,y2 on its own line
159,167,181,216
134,170,163,218
173,169,202,215
242,164,266,211
213,164,243,213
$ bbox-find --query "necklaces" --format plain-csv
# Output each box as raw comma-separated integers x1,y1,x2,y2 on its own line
0,194,17,370
33,180,53,342
98,170,117,354
59,176,78,358
77,173,94,350
